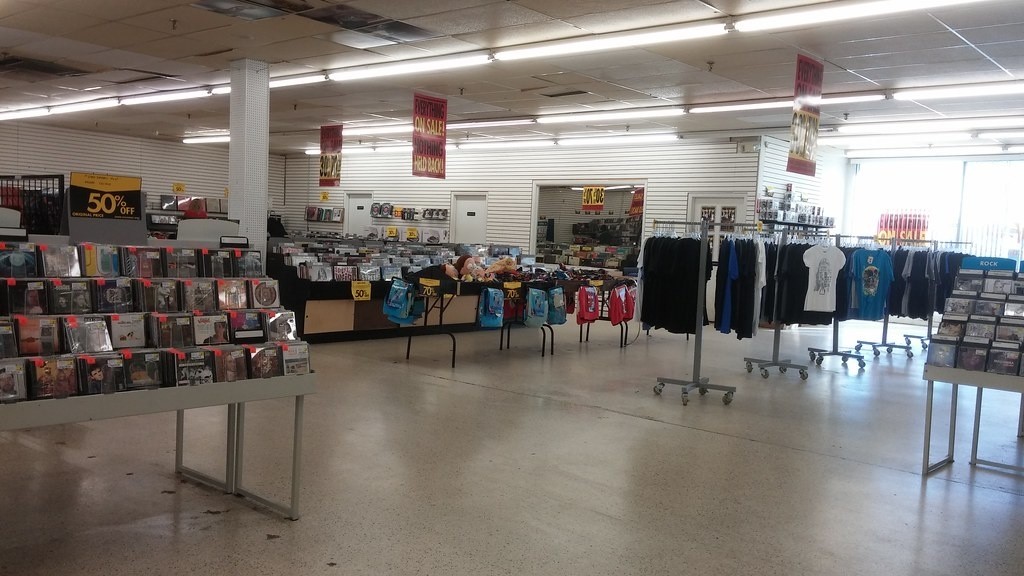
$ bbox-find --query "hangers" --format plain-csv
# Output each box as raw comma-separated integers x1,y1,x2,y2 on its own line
649,218,976,256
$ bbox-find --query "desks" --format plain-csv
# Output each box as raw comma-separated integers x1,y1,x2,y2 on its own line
0,369,316,522
920,363,1024,476
399,266,638,368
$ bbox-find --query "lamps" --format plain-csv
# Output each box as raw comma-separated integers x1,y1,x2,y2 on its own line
0,0,1024,158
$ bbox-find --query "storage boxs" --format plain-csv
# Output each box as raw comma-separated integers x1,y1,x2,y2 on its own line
159,194,228,214
759,191,835,226
364,226,448,244
